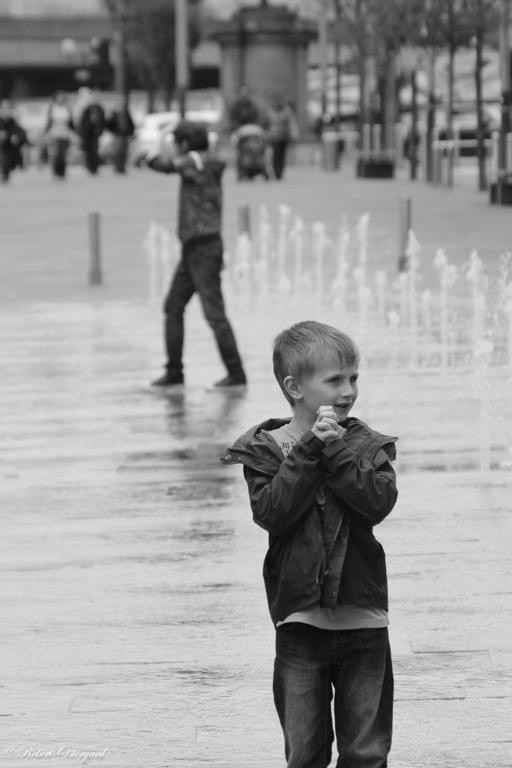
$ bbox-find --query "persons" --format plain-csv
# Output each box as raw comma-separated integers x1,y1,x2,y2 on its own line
1,86,135,183
228,83,299,180
219,318,405,768
129,116,249,390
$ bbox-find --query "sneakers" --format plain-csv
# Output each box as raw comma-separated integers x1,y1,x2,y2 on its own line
152,373,184,386
216,376,247,386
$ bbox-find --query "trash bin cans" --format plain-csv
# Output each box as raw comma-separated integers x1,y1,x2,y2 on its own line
321,134,344,171
